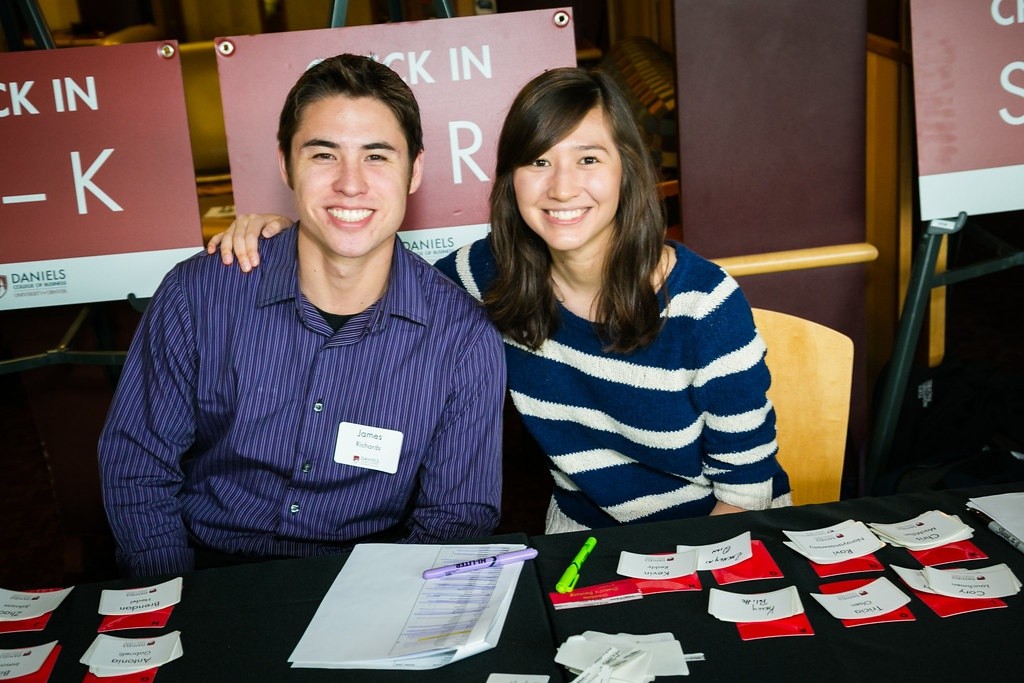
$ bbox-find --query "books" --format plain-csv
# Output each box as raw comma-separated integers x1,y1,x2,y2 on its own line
289,544,525,670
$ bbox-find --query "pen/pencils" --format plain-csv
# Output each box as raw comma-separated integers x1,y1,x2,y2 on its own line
972,509,1024,555
422,547,538,581
556,536,598,594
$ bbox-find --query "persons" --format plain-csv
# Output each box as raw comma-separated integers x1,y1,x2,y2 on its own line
207,67,792,535
97,50,506,582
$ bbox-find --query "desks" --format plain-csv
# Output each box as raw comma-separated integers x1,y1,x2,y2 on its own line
0,487,558,682
533,490,1023,683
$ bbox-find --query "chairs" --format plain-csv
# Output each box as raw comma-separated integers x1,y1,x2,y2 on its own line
750,308,853,502
